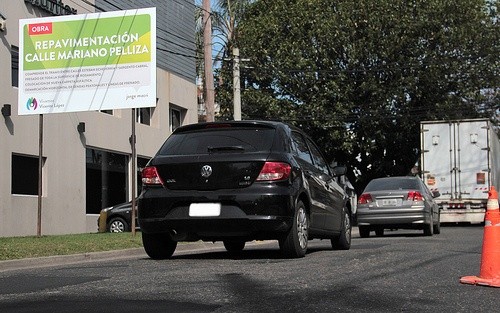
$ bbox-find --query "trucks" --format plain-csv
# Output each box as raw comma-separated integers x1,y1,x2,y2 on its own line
420,118,500,224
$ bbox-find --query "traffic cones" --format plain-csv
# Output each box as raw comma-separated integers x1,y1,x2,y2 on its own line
460,186,500,287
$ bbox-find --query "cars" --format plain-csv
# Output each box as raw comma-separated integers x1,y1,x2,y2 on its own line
137,119,352,259
356,176,443,237
97,196,140,234
